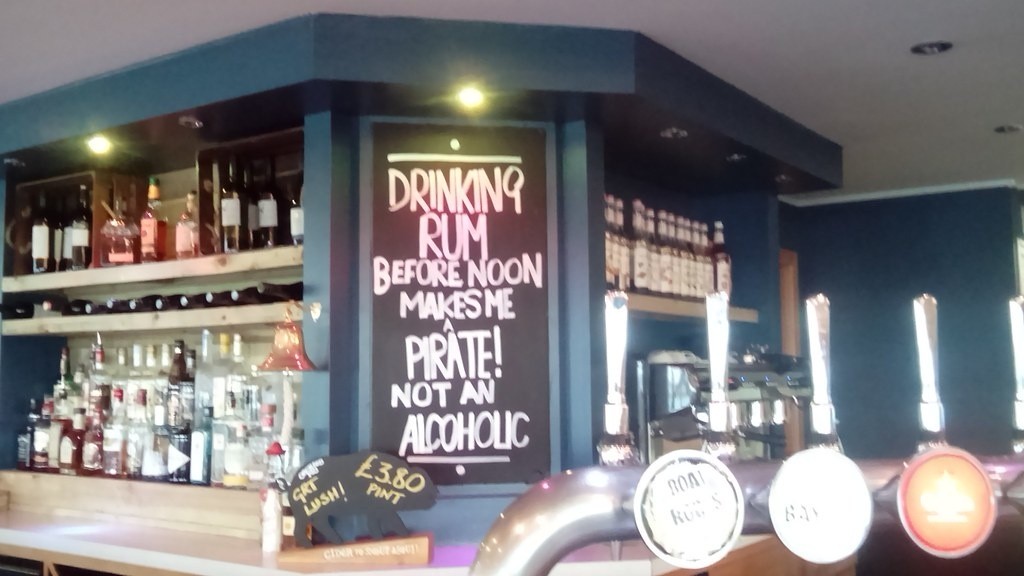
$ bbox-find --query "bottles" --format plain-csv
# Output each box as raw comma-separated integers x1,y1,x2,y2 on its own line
16,328,262,491
4,157,304,318
603,192,732,303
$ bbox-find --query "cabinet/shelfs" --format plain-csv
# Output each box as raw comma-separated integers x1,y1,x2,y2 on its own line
0,120,308,489
604,176,787,464
